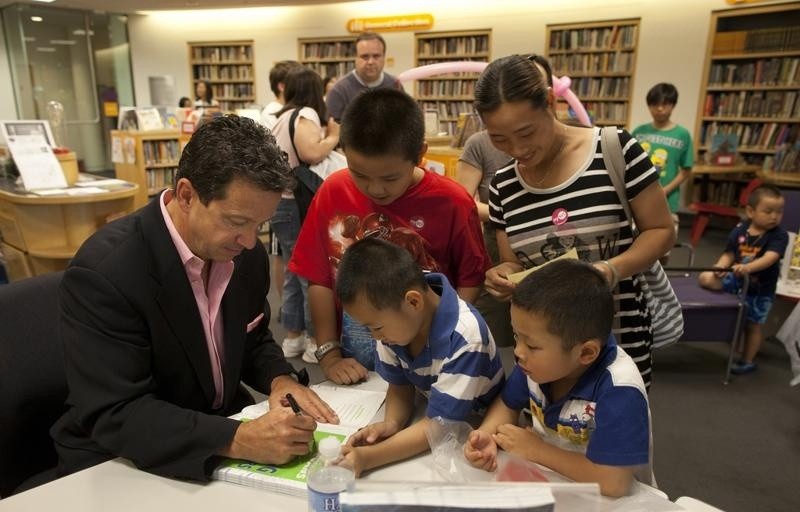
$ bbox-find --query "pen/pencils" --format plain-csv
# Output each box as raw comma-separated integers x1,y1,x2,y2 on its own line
286,393,317,448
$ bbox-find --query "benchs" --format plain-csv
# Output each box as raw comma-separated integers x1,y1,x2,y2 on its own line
690,203,745,250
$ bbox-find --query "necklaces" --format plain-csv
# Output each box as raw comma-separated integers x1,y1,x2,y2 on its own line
524,126,567,188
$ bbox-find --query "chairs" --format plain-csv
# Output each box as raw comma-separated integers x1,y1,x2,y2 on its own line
658,242,749,387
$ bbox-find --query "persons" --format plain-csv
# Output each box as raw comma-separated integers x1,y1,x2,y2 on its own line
179,97,191,110
286,87,493,384
49,113,340,485
324,31,403,123
699,186,789,374
322,76,336,103
473,54,677,403
331,238,506,478
463,259,659,498
630,82,693,260
259,59,300,130
268,65,341,363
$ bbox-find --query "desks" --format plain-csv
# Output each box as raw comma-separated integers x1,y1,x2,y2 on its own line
692,164,760,204
1,367,691,512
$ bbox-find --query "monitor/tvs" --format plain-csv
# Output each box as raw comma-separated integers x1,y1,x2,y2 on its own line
0,120,56,149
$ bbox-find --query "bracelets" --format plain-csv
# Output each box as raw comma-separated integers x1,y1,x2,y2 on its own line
313,339,344,361
596,259,619,290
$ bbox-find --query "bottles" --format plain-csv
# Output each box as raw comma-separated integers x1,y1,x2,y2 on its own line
306,437,357,512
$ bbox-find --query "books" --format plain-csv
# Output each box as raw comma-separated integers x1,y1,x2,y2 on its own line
338,484,555,512
549,24,637,125
143,140,180,191
190,45,254,112
417,36,489,150
302,41,356,81
211,385,386,500
694,27,800,208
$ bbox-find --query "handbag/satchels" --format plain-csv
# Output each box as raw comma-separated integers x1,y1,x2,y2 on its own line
600,126,685,353
289,104,349,224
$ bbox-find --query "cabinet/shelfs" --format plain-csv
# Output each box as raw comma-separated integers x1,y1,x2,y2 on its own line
0,170,140,286
188,39,257,114
683,1,800,210
112,127,193,216
544,16,641,130
412,27,493,134
297,36,356,83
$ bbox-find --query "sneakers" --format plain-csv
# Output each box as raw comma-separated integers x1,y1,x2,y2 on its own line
281,330,308,358
730,359,758,375
302,343,319,364
